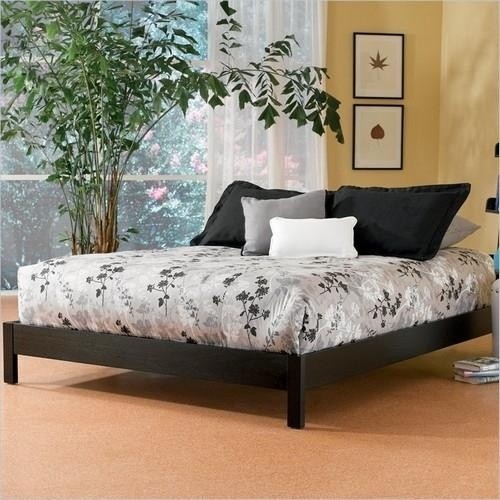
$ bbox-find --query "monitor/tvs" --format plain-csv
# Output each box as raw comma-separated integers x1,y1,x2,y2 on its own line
453,373,500,385
452,356,499,371
451,368,499,377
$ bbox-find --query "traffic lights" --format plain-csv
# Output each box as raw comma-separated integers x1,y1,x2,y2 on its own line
190,181,481,261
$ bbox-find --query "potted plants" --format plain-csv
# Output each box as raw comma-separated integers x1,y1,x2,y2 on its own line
353,104,404,170
353,32,404,99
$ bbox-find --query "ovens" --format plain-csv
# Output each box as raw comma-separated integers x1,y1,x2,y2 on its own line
3,246,497,429
